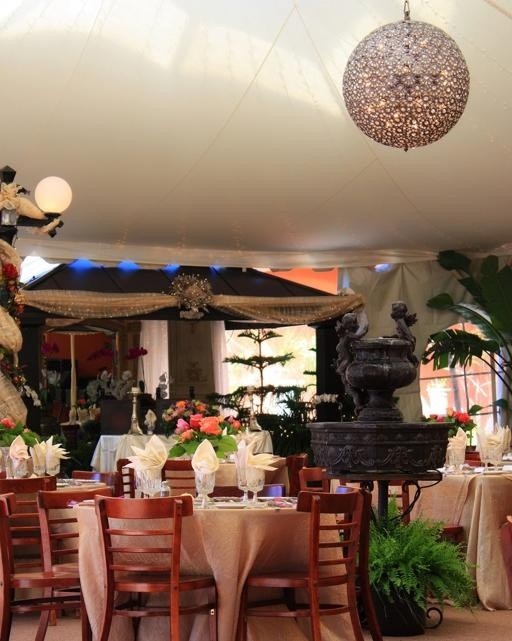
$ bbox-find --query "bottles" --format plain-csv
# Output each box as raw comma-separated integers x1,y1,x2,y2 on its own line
161,481,171,497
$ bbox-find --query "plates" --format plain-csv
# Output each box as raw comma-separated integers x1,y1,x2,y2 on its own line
213,503,247,509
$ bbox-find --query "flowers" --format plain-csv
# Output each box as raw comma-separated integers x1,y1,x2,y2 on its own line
420,408,478,439
0,417,40,447
160,398,241,459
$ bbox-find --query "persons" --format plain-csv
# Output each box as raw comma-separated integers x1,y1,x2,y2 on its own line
386,299,422,367
334,310,363,377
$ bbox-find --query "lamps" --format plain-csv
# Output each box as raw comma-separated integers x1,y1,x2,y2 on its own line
342,1,470,151
19,177,73,237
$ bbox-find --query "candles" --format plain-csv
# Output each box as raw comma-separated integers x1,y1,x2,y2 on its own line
131,387,140,393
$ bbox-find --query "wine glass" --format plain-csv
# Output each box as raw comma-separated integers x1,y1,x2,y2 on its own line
194,471,216,510
236,465,265,508
447,445,504,475
134,469,162,498
2,448,61,479
